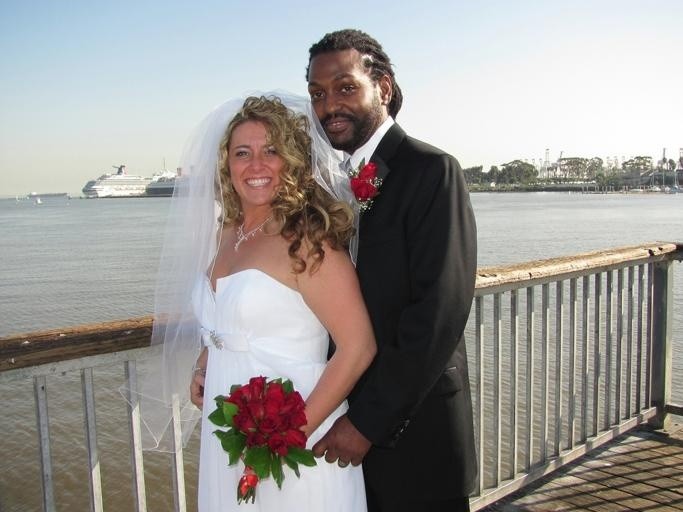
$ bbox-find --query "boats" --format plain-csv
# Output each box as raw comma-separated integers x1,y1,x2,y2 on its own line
82,158,189,197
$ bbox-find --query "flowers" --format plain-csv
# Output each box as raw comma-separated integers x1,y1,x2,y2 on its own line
212,377,316,504
348,161,380,201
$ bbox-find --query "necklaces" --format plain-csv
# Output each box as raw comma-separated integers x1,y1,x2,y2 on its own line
231,214,272,253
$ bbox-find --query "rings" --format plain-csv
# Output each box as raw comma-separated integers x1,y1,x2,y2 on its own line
338,459,348,467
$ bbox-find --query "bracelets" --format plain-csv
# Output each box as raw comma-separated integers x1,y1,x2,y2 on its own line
190,365,205,378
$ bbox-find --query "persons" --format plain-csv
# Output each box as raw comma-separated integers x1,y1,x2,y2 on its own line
115,88,384,512
189,28,483,512
